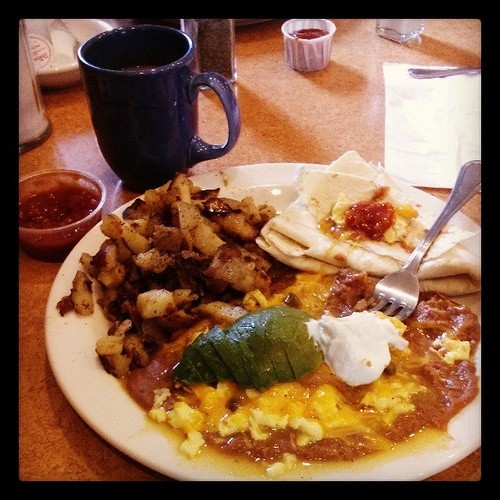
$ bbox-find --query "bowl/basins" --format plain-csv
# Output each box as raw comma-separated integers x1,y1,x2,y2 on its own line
19,167,106,263
21,20,117,90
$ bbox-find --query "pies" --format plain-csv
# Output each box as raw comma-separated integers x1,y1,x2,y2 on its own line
255,150,481,295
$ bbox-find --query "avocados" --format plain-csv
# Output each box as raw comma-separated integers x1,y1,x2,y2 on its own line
173,306,324,390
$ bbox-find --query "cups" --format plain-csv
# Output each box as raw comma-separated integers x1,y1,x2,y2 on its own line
278,18,337,72
180,20,240,86
18,23,53,156
78,23,242,192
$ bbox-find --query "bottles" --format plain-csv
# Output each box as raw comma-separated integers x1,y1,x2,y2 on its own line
375,19,426,43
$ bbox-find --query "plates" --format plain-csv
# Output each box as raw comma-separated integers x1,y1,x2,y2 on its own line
44,163,482,481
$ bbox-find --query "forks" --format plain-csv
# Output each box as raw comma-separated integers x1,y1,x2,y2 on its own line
362,158,480,323
